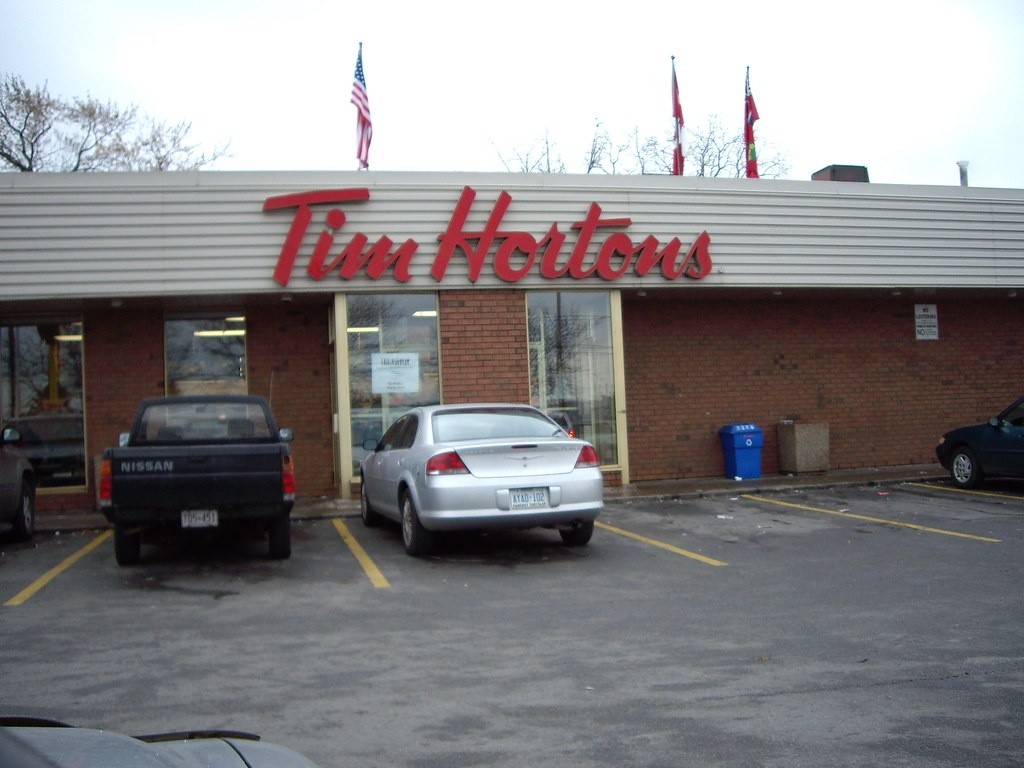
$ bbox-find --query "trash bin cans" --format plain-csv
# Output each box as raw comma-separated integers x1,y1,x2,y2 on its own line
720,423,764,481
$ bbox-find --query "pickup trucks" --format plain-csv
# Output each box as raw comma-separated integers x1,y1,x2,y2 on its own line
99,394,296,564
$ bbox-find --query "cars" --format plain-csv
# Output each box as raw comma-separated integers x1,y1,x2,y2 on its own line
547,411,576,437
351,407,410,474
0,424,36,542
360,402,605,555
936,396,1024,489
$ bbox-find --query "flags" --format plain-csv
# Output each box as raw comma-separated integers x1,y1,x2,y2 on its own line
744,71,763,180
669,63,684,177
348,47,373,169
